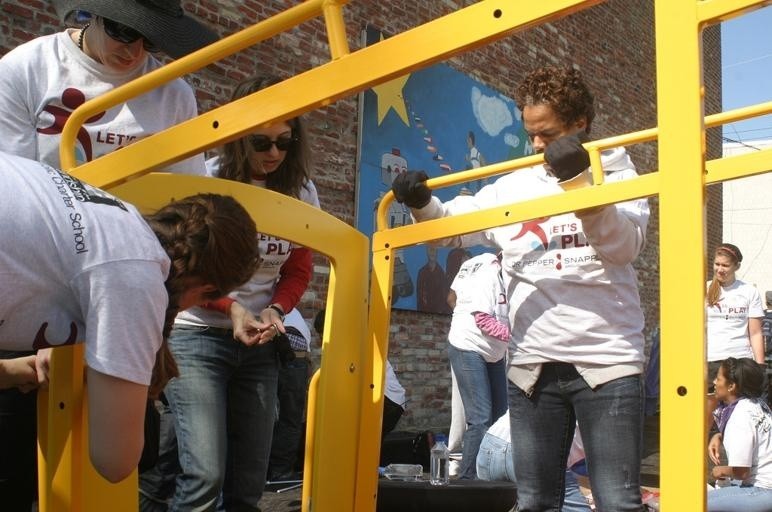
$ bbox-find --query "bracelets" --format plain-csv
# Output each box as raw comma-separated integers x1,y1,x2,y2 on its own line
758,364,766,373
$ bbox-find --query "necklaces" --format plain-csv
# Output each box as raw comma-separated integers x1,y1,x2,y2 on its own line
79,24,90,50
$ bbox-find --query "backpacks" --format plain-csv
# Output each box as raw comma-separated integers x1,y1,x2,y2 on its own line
761,308,772,356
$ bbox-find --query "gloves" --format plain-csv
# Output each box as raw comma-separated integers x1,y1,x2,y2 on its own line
543,127,590,177
392,171,431,209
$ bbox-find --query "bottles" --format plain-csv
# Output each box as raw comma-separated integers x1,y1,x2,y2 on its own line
377,464,424,482
429,433,451,485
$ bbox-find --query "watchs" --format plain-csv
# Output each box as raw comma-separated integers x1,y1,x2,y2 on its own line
264,305,285,323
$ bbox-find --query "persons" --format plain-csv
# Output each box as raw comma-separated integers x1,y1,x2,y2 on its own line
708,357,771,510
1,152,260,510
706,244,765,438
445,251,514,482
136,304,407,511
391,67,650,512
2,2,219,510
156,74,320,510
476,408,591,510
447,253,497,481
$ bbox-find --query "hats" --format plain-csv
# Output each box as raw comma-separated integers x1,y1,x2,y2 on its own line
53,1,220,59
766,291,772,304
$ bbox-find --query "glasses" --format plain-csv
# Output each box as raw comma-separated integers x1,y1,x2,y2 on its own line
103,19,161,53
249,134,300,151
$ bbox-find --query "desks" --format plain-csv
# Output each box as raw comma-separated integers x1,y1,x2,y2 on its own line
377,472,518,511
